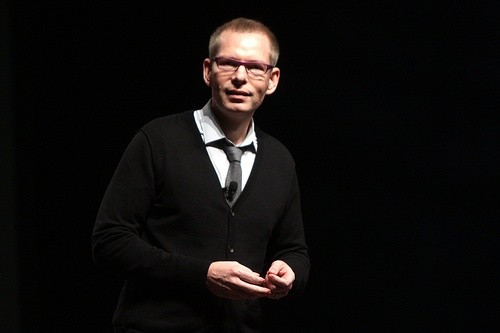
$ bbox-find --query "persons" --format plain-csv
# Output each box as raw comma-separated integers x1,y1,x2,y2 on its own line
97,18,311,333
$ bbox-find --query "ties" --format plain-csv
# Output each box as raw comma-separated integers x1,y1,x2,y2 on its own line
214,138,253,209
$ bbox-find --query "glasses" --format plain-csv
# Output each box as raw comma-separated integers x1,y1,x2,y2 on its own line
211,56,274,78
